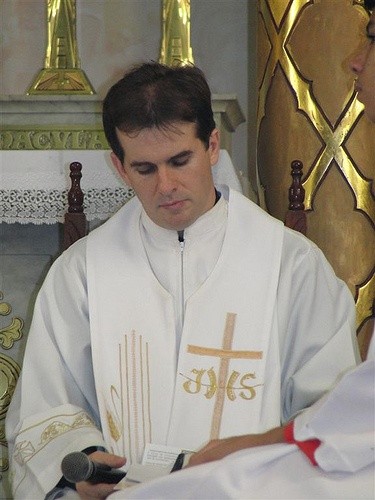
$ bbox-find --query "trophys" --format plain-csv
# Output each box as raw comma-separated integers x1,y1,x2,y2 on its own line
26,0,97,95
158,0,195,70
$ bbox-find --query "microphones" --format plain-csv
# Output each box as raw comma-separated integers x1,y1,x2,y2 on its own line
61,451,128,484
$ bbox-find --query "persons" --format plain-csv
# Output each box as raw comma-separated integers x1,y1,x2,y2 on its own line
105,0,375,500
5,60,362,500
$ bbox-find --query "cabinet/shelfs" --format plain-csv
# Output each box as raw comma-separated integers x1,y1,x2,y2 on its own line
0,94,246,500
